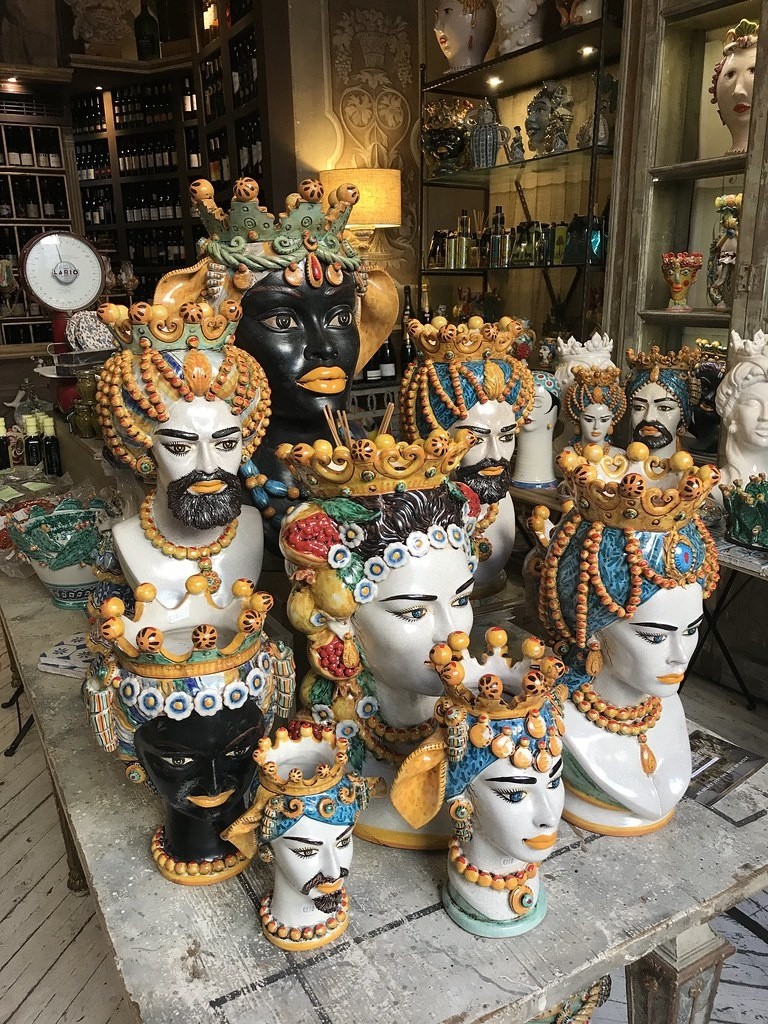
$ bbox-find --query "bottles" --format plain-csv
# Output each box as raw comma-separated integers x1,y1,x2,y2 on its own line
380,338,397,382
134,0,160,62
589,203,602,265
491,206,505,235
401,334,417,377
421,283,434,324
0,175,69,218
0,227,70,268
401,285,416,329
0,95,69,168
0,418,12,470
365,350,382,384
603,195,610,235
201,31,263,192
458,208,471,238
70,79,202,306
22,412,63,477
0,272,53,343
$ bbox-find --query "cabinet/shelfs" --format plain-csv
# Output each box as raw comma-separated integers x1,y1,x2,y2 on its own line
0,0,271,361
418,0,768,465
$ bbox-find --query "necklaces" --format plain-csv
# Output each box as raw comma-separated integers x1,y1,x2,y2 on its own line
151,819,248,877
570,681,662,774
138,484,239,592
259,888,349,941
448,829,539,915
357,715,438,773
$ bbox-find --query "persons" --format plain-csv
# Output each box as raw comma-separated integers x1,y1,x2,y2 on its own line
86,0,768,949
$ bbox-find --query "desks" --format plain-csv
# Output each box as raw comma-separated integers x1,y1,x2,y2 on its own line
0,453,768,1024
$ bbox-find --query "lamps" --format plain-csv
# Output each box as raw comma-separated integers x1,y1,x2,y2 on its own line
320,168,403,273
